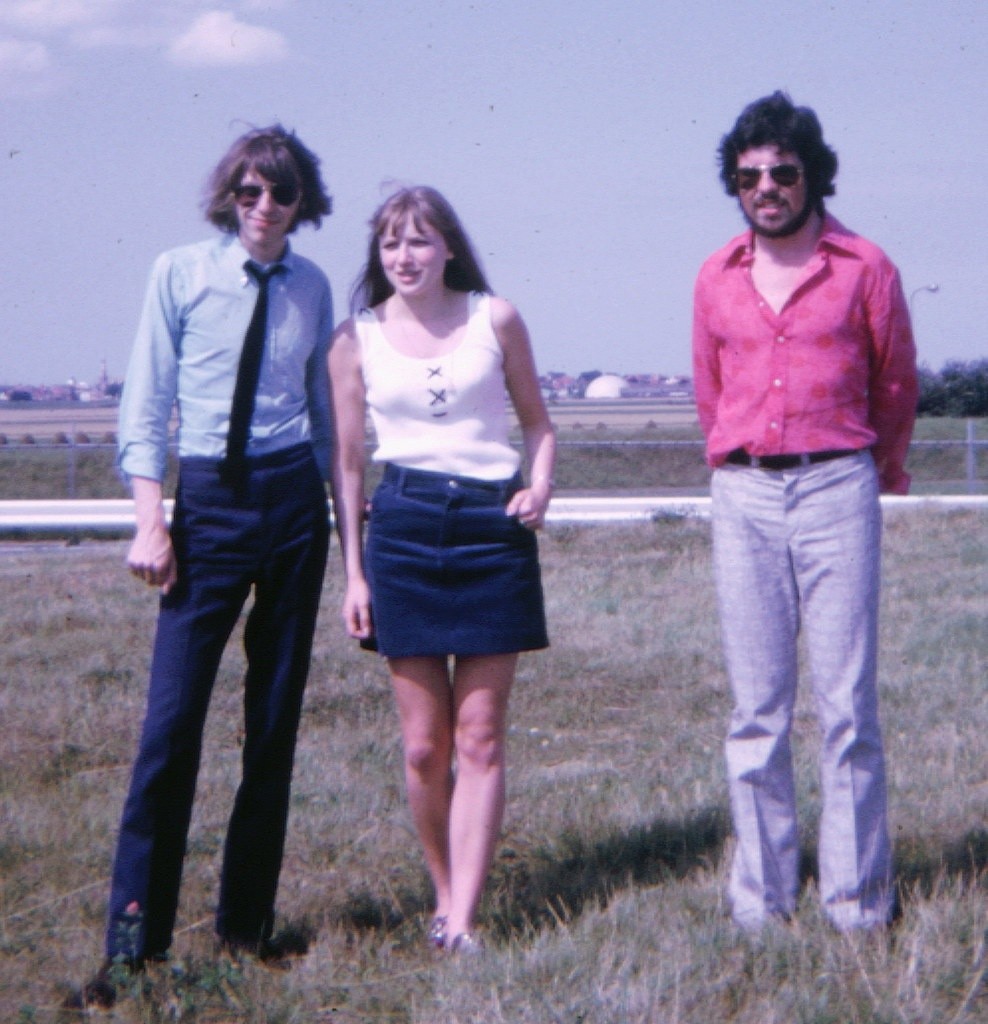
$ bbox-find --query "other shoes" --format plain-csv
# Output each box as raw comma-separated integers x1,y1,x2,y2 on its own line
221,936,290,971
64,960,153,1010
430,916,484,955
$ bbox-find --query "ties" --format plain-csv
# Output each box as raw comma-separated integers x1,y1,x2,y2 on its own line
219,261,282,488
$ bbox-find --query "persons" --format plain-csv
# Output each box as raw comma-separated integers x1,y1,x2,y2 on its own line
692,90,919,952
57,124,335,1023
326,186,557,958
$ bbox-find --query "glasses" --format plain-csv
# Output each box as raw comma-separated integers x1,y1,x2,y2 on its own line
233,181,302,206
730,164,808,190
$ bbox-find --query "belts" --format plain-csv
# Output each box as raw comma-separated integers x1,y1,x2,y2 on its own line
726,452,854,471
